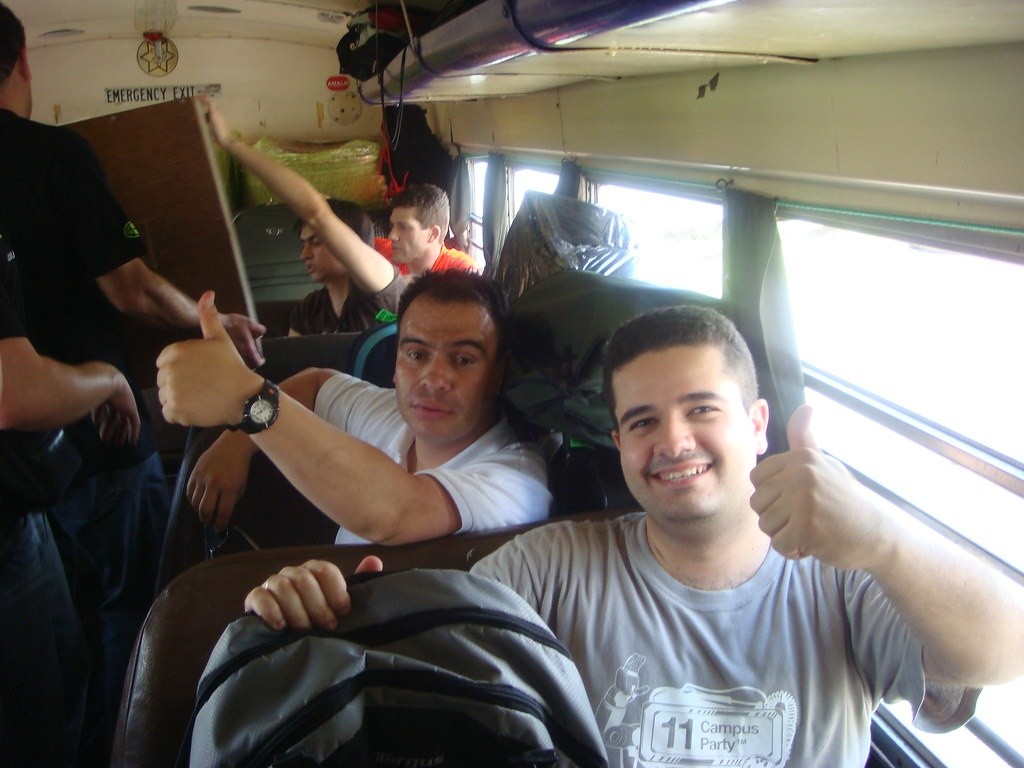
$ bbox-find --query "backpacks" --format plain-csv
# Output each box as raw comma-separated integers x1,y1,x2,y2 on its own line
375,104,445,195
337,5,438,83
172,569,612,768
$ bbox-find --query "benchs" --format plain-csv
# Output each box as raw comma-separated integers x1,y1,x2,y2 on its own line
106,323,645,768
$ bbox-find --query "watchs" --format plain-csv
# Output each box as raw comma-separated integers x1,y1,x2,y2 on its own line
239,378,280,434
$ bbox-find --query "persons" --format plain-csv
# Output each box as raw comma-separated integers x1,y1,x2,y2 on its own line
197,92,483,338
0,2,266,768
156,266,1024,767
